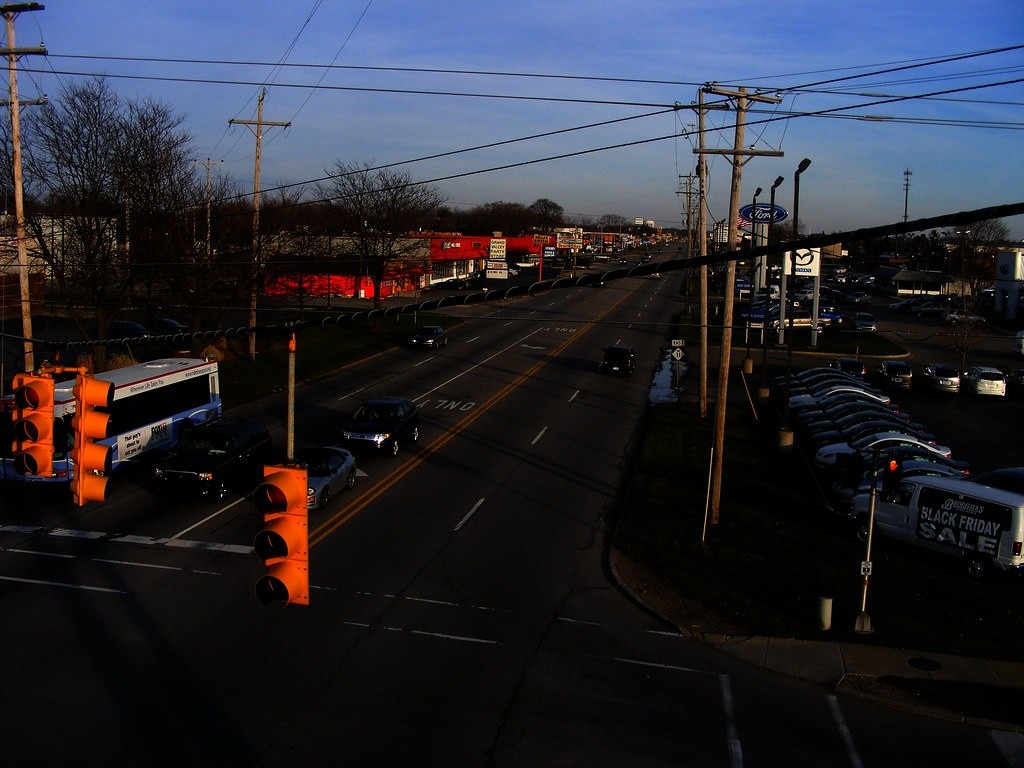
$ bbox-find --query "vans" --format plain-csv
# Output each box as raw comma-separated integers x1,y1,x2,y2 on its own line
848,476,1024,577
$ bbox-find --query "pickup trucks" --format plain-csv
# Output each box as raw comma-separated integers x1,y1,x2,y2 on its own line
765,310,832,336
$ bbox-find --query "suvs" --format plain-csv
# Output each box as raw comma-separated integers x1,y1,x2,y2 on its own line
152,416,273,503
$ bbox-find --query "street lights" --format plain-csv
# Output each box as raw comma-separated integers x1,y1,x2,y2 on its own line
570,225,580,277
750,158,811,360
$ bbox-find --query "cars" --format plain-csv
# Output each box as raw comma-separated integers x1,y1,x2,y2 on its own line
619,249,663,280
837,358,866,383
854,460,976,495
90,319,193,356
408,326,448,349
291,398,418,510
436,279,472,291
775,367,939,451
833,446,972,488
887,298,985,328
35,349,93,370
733,264,877,335
814,431,953,469
878,360,1006,397
971,467,1024,495
601,345,638,377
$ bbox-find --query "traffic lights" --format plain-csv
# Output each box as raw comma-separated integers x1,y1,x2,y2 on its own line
9,371,115,507
251,464,308,611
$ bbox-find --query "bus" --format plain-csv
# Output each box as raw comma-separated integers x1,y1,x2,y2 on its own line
0,358,221,482
